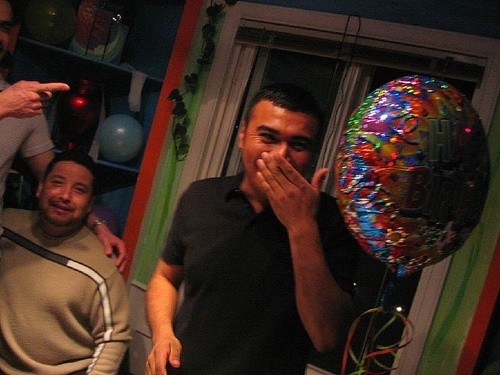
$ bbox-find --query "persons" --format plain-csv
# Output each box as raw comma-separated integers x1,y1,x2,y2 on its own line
142,79,362,375
0,146,133,374
0,0,130,276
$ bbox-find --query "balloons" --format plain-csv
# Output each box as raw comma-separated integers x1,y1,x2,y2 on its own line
23,0,78,45
94,112,146,164
335,72,494,280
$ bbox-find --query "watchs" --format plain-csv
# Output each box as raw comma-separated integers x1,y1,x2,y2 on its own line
92,216,106,230
